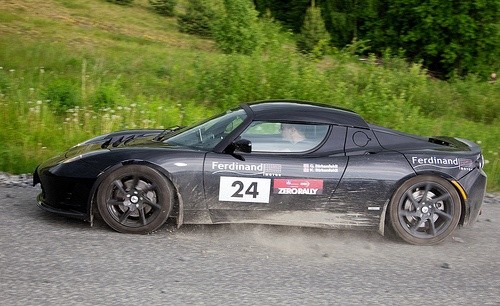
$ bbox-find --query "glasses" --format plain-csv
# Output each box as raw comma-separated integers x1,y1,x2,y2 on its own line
281,126,289,129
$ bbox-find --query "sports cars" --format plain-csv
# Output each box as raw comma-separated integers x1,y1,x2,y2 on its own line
32,100,488,247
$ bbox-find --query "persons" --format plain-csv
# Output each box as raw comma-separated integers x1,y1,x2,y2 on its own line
279,122,313,152
